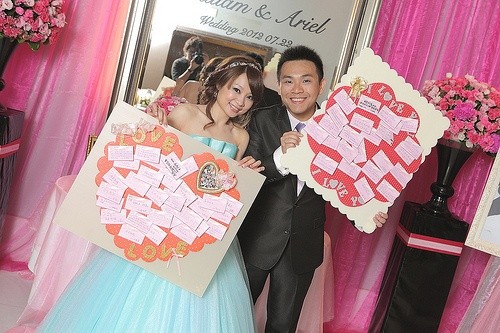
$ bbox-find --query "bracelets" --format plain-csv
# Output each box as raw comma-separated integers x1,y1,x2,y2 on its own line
186,67,194,74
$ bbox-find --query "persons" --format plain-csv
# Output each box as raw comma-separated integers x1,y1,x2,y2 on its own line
171,56,225,104
236,44,390,333
247,52,282,108
84,54,265,333
170,36,209,85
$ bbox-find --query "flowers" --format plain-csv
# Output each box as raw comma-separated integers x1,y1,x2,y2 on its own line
418,71,500,154
155,96,188,116
0,0,69,53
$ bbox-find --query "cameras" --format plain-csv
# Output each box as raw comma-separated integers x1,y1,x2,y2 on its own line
192,52,203,64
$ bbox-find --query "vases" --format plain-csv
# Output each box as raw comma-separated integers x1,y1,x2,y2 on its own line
420,138,479,217
0,33,20,92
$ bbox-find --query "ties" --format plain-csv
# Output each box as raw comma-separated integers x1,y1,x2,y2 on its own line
295,122,306,133
